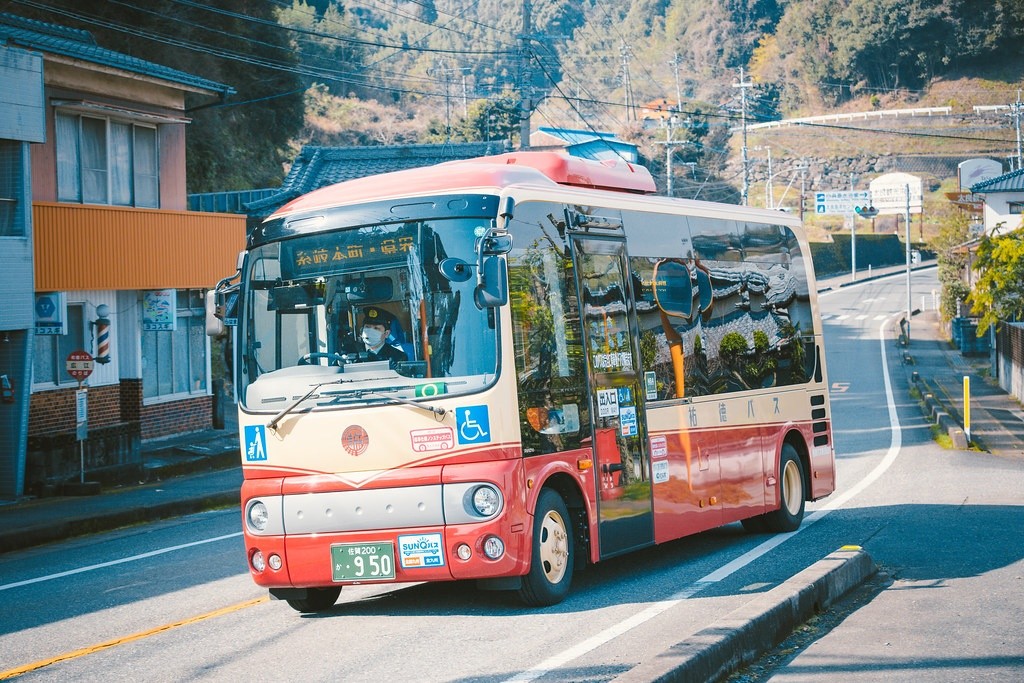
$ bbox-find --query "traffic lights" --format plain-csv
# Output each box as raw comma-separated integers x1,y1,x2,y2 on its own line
855,206,877,215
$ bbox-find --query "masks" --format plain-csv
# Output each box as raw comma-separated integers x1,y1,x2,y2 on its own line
362,328,386,346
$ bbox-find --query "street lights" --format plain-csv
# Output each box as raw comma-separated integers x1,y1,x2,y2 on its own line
765,165,809,209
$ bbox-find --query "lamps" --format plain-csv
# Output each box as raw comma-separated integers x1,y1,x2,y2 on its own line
88,303,111,366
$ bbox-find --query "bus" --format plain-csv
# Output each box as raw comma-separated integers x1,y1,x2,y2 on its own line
206,150,838,613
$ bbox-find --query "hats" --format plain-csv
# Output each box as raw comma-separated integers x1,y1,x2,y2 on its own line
361,307,397,325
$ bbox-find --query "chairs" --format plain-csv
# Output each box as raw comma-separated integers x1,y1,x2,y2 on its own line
385,320,414,361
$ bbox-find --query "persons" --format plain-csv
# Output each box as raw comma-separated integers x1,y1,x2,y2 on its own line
335,306,409,368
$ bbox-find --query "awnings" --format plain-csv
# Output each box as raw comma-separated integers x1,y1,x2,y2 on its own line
944,191,984,212
